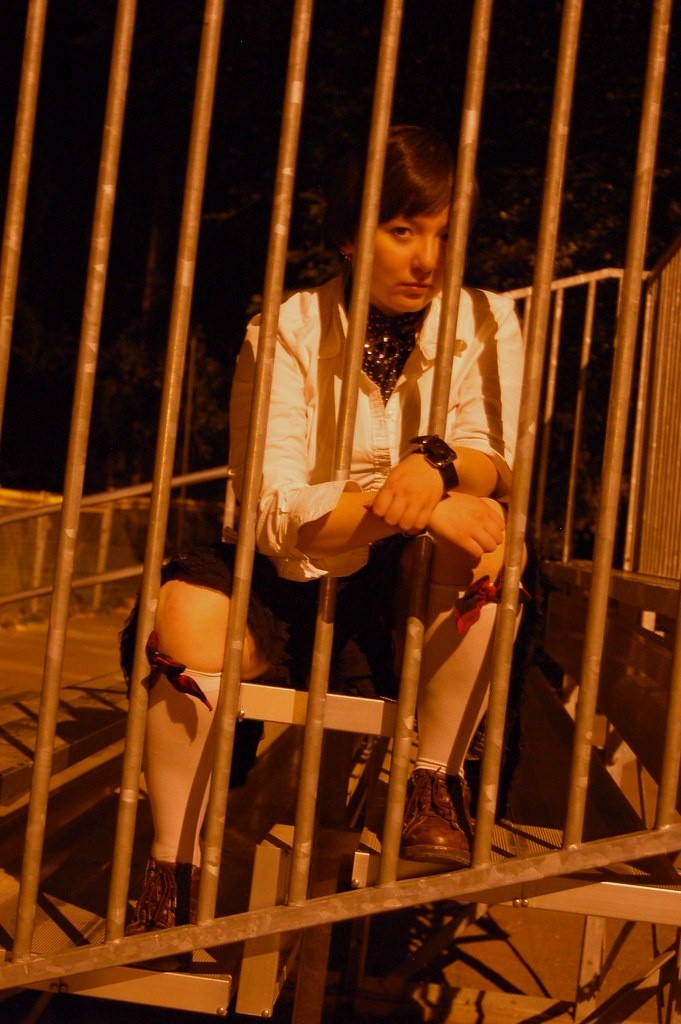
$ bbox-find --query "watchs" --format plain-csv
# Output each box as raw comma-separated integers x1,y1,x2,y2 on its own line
401,434,458,491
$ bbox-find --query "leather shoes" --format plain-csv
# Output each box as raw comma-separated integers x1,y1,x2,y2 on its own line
401,767,474,866
122,853,201,972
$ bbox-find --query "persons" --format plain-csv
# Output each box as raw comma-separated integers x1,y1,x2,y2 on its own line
123,124,525,971
